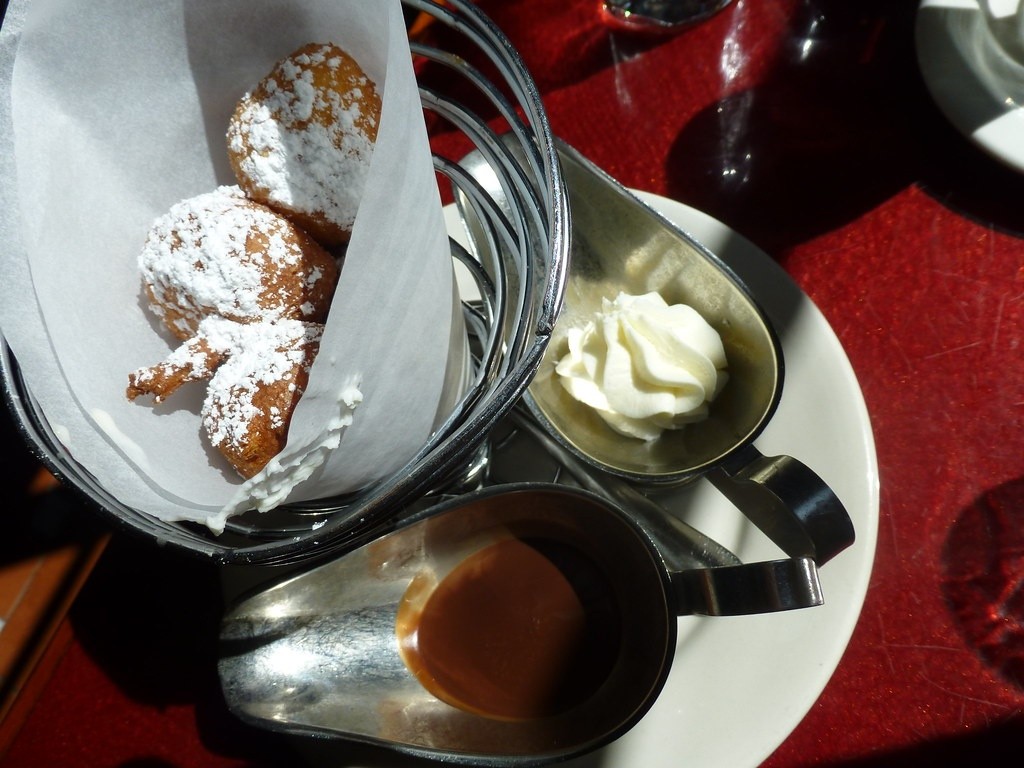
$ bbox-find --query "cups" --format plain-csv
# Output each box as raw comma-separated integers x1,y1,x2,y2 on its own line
454,129,856,570
215,482,825,768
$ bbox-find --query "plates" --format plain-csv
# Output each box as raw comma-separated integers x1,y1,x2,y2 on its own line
912,0,1024,177
214,182,882,767
